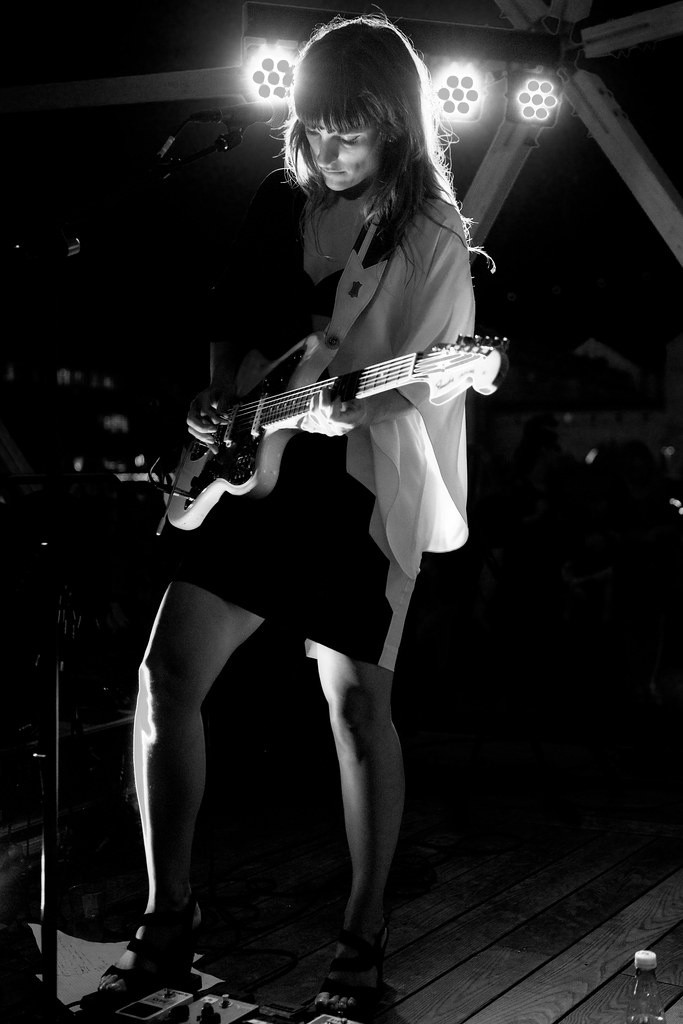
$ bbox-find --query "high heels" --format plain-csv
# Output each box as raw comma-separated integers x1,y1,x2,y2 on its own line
97,882,203,996
313,915,393,1017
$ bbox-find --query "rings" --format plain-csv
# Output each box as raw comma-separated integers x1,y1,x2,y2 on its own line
20,858,33,870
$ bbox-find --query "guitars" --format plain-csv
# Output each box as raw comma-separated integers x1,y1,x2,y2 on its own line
161,330,511,532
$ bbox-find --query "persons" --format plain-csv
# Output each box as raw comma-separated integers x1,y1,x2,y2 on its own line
468,415,683,705
0,844,33,928
80,20,474,1015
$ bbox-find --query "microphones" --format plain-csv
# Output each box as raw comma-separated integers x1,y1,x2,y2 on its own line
190,97,288,126
